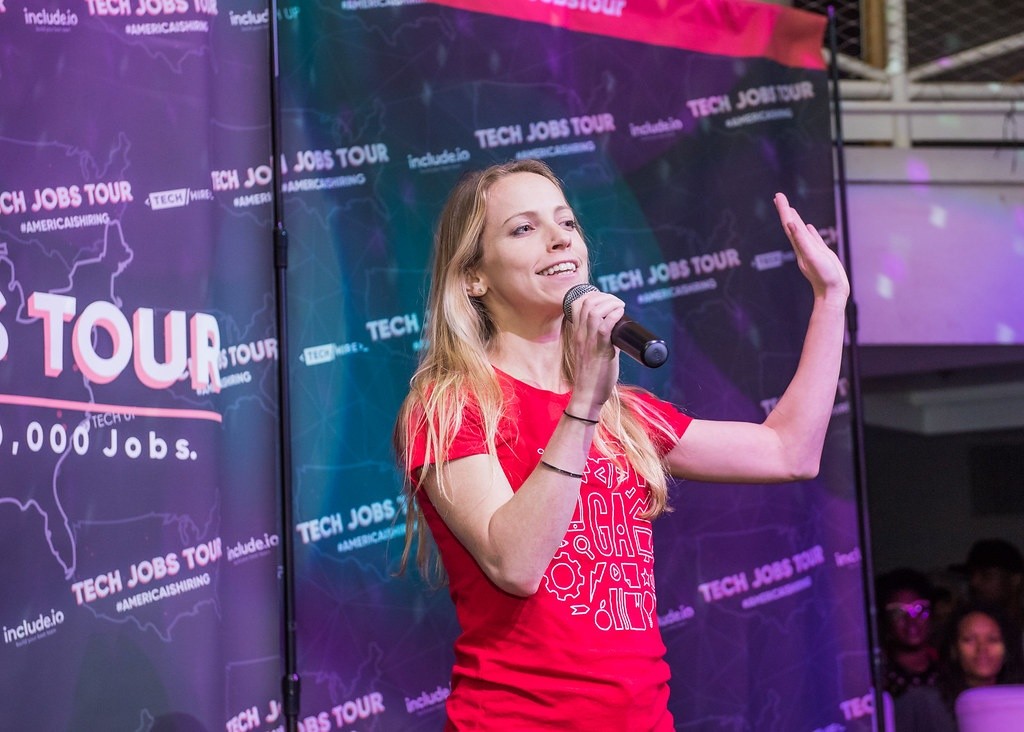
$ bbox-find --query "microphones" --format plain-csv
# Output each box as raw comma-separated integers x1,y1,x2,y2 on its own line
561,283,671,370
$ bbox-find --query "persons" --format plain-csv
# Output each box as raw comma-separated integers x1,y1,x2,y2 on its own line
394,161,849,732
876,536,1024,732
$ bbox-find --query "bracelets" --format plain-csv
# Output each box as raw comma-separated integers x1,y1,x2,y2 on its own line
563,409,600,423
539,459,583,478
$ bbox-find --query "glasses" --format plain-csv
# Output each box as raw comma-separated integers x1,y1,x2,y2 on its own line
885,600,933,623
